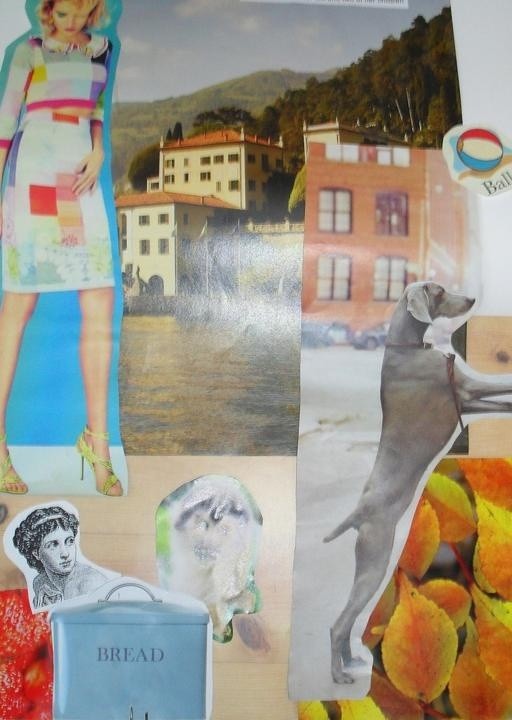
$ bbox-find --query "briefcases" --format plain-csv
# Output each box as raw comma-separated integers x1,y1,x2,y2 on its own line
50,583,208,720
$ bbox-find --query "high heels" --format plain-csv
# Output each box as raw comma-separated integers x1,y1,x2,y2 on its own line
1,433,28,494
79,426,124,496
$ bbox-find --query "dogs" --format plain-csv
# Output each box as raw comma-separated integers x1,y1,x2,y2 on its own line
323,280,511,685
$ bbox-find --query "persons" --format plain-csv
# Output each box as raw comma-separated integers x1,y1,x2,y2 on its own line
14,505,109,610
0,0,125,501
